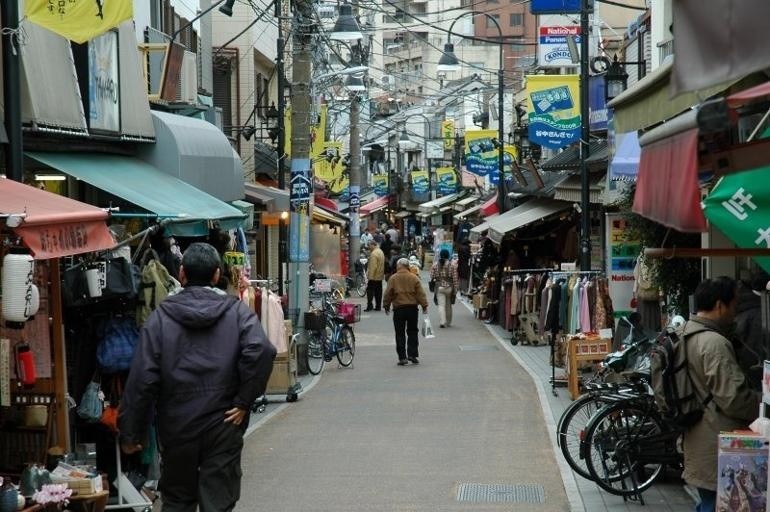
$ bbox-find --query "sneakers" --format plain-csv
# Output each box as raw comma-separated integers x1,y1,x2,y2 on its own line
363,307,382,312
397,359,408,364
408,356,419,364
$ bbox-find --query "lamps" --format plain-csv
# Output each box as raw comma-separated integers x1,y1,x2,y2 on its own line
224,125,256,141
603,54,646,104
173,0,235,40
265,102,280,144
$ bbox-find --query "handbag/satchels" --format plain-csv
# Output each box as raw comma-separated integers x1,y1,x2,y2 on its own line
422,313,436,337
61,256,175,430
429,278,438,291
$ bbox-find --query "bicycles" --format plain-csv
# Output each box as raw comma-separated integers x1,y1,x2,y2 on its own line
355,254,368,297
557,354,684,504
303,262,361,374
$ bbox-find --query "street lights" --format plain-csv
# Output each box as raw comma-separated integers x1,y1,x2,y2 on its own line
276,2,363,294
439,10,506,214
398,113,432,226
365,125,395,194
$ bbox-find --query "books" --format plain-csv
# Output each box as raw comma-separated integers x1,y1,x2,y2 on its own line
715,426,767,512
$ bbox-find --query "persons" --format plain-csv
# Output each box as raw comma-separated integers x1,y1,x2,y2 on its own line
118,243,277,511
361,221,433,312
383,258,428,365
679,274,760,512
726,268,769,392
430,250,459,327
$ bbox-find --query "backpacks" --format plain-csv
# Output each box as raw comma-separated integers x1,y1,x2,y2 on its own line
649,323,714,427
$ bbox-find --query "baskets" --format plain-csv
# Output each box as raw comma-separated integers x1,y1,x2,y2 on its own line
304,311,327,329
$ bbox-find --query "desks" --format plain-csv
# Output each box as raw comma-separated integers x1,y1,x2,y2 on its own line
568,338,612,401
